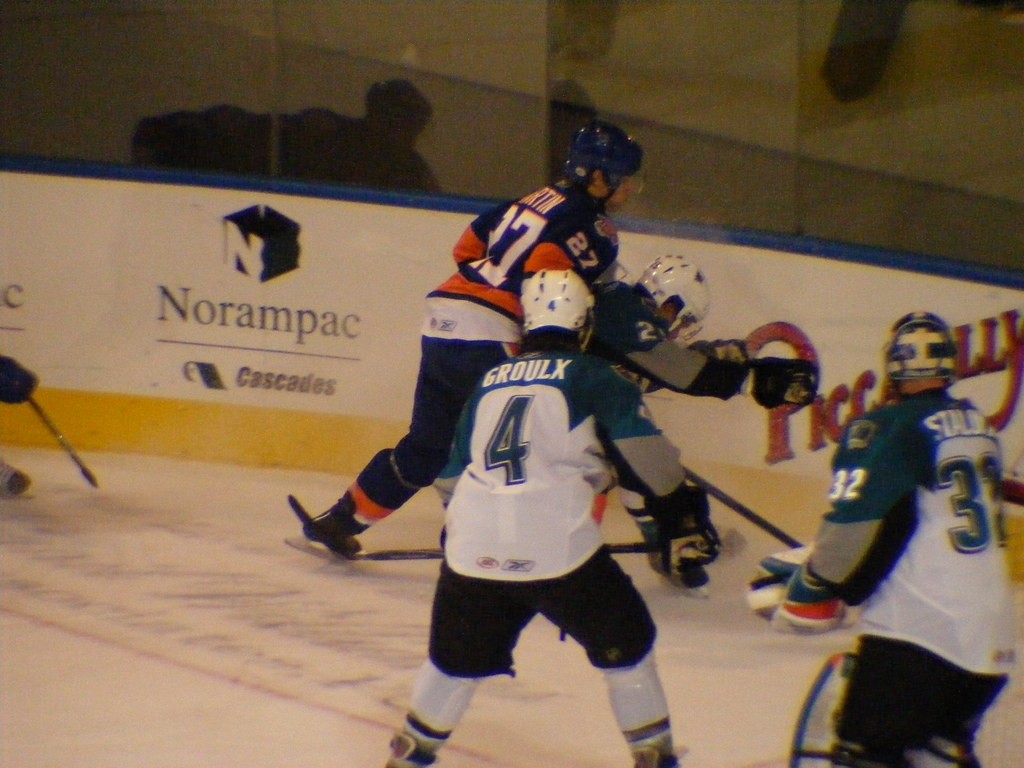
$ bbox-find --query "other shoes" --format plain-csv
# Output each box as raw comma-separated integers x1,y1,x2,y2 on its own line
0,463,32,500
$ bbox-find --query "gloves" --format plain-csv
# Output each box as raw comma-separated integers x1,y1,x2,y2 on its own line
643,483,721,574
770,553,851,635
0,356,34,405
749,356,818,408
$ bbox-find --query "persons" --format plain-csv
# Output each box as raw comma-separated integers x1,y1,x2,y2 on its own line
129,79,438,194
385,269,723,768
1,354,40,496
303,122,820,588
746,310,1014,768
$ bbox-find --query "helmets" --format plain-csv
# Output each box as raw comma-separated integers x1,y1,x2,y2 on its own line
635,254,711,342
886,311,957,391
563,122,644,200
519,268,595,355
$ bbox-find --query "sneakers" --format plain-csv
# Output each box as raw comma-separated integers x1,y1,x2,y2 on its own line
284,490,367,567
635,521,712,599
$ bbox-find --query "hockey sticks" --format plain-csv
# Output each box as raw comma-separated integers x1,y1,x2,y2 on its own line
286,491,664,563
682,462,805,549
28,395,100,488
